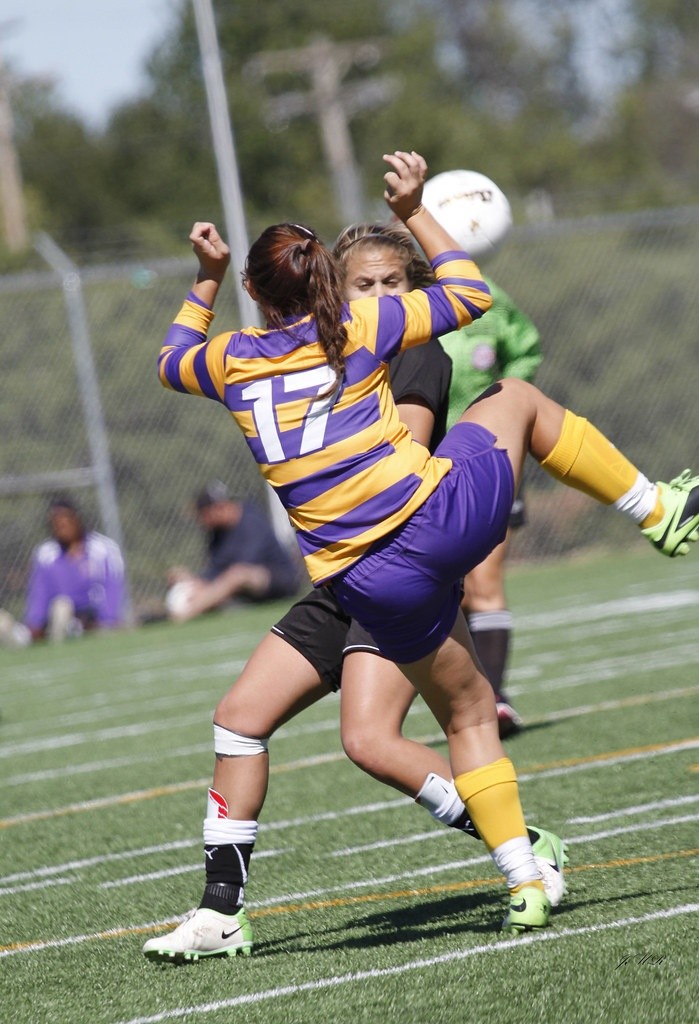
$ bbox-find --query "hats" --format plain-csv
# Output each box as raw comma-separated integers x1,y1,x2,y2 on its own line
196,479,243,510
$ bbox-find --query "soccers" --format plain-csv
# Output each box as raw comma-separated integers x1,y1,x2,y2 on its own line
417,169,519,266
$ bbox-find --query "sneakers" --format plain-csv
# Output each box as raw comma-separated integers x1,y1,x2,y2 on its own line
524,825,569,907
142,906,253,961
495,702,523,736
641,474,699,557
501,887,551,935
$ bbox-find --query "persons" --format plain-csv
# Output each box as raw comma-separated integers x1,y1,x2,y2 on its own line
1,497,135,645
386,217,543,737
162,484,295,620
143,223,576,966
154,149,699,934
